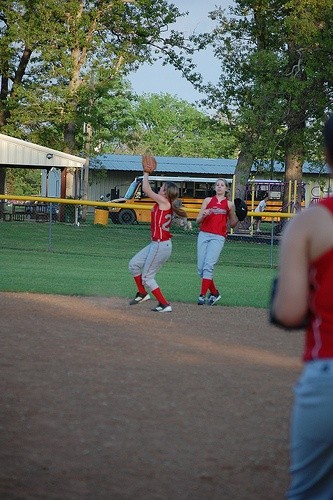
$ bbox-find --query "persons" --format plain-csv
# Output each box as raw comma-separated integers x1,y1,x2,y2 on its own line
269,115,333,500
249,195,268,232
128,164,188,312
196,179,248,306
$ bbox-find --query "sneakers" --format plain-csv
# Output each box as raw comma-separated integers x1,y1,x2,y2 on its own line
197,294,206,305
151,304,172,311
208,290,221,306
129,292,150,305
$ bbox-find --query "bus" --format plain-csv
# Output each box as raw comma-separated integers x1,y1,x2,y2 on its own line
107,175,308,226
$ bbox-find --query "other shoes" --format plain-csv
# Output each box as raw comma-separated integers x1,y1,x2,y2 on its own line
249,226,252,231
257,230,261,232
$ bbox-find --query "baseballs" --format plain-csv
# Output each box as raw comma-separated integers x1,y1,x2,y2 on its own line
240,206,246,212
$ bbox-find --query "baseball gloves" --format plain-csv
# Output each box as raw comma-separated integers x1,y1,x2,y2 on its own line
234,197,248,222
142,154,157,174
271,277,312,330
